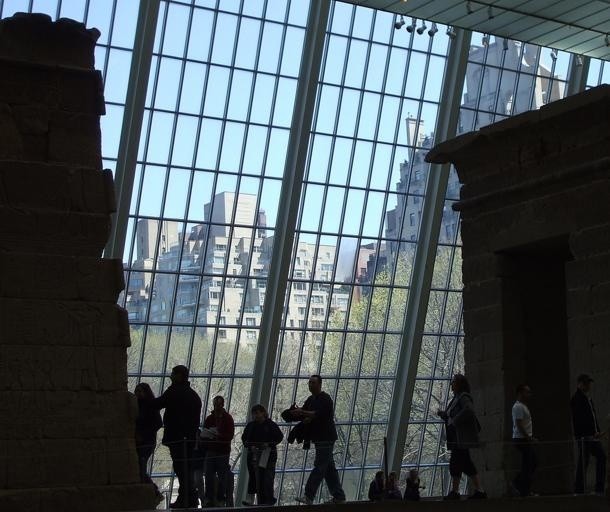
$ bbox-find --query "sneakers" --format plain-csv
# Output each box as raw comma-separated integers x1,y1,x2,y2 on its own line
467,487,489,501
323,497,346,505
507,481,520,498
441,491,462,503
523,489,539,499
295,493,315,505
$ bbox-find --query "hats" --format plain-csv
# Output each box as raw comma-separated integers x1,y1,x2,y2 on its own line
577,373,595,384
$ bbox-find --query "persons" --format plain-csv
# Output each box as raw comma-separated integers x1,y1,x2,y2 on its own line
569,373,600,497
368,470,387,502
134,382,164,510
403,468,427,502
132,364,203,509
241,404,284,504
203,395,236,509
436,373,489,505
387,470,403,502
505,384,545,497
292,374,348,506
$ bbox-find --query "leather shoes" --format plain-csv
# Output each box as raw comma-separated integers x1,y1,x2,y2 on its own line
168,499,200,510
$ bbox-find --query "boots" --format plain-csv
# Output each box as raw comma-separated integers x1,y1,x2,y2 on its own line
242,492,255,506
